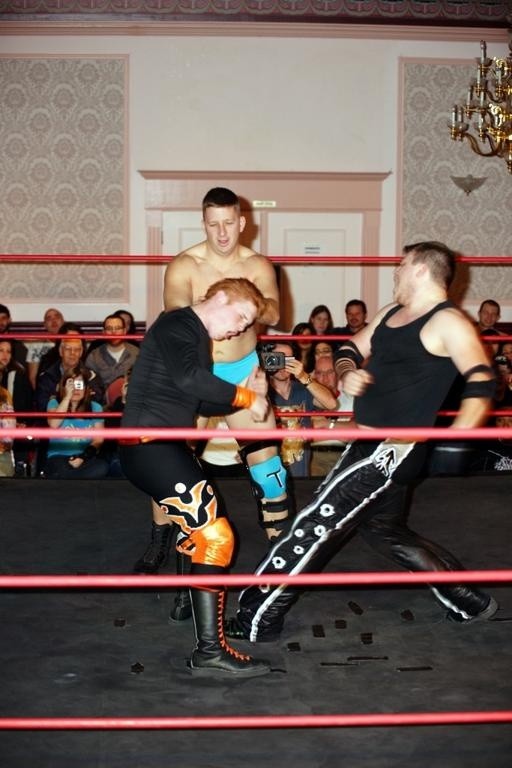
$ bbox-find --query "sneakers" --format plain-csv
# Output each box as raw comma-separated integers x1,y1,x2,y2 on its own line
223,617,256,641
442,597,497,626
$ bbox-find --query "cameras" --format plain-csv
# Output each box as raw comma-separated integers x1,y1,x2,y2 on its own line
73,380,83,389
261,351,285,372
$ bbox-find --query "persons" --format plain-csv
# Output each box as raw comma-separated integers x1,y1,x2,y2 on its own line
0,300,139,479
260,299,511,480
223,242,499,643
120,279,271,678
134,187,289,576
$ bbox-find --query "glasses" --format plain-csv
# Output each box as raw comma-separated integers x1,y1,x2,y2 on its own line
314,348,331,355
63,346,81,353
105,326,123,331
318,370,334,376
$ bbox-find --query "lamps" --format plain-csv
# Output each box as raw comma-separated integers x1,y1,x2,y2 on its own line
449,28,512,172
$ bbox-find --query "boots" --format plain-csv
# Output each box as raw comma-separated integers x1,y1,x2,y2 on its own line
169,550,193,624
141,522,172,570
189,586,272,679
